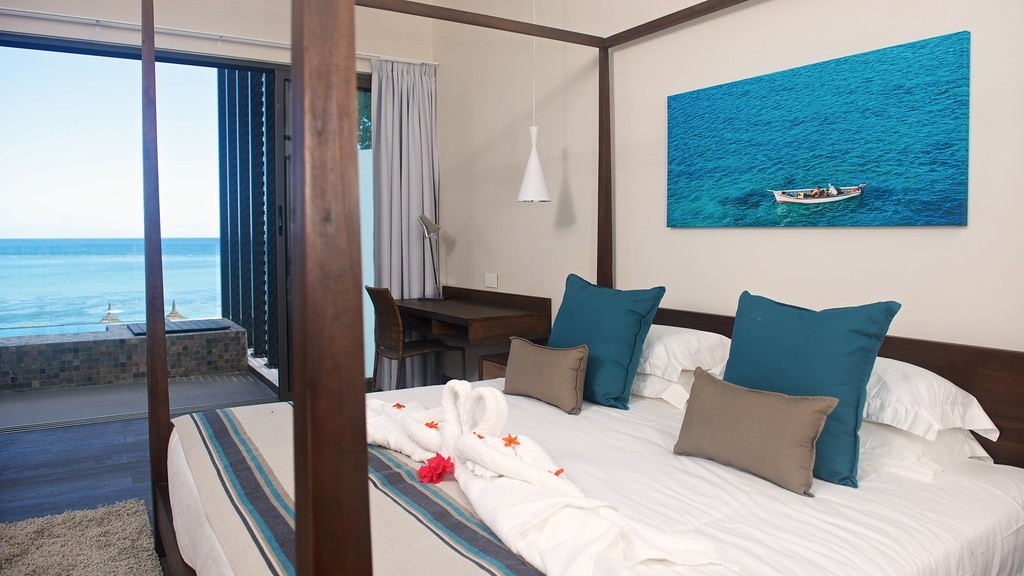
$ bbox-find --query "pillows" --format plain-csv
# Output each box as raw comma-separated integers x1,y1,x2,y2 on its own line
636,323,732,381
547,274,666,410
630,373,724,411
724,289,902,490
865,356,1000,444
673,367,839,498
858,420,993,480
502,335,589,416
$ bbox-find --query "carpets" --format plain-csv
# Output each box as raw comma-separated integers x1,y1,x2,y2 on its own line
0,496,165,576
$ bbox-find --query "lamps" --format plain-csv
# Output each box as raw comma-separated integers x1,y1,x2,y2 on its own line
418,214,448,301
517,0,554,203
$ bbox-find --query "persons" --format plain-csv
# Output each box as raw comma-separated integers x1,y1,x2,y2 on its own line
811,183,838,198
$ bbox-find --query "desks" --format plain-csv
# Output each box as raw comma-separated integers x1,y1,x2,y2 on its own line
396,286,551,382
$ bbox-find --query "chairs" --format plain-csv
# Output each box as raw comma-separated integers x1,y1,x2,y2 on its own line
364,285,466,389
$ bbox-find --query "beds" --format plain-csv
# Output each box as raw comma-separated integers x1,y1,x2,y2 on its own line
166,307,1023,576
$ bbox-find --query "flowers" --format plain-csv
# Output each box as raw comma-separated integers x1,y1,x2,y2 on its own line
416,452,454,485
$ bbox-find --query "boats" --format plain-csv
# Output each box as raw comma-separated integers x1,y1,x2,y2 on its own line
774,182,867,204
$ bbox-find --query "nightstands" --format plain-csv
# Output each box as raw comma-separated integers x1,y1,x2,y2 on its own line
478,353,509,381
